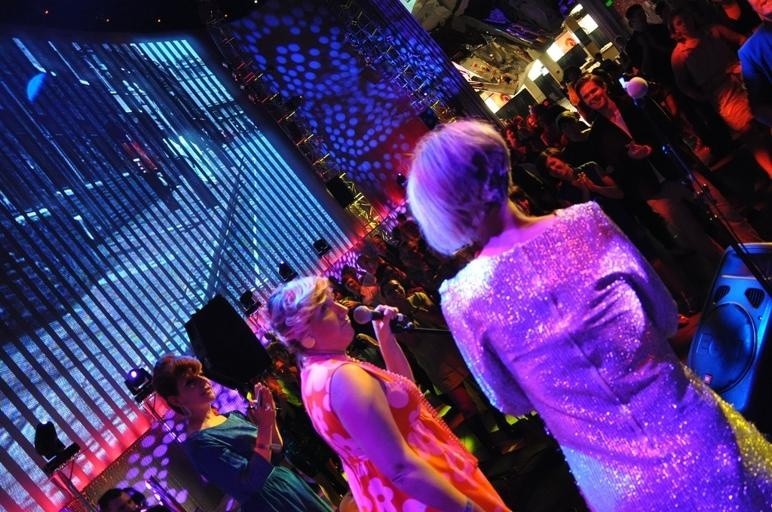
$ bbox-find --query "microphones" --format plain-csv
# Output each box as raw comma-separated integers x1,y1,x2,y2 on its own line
354,306,407,324
627,77,658,99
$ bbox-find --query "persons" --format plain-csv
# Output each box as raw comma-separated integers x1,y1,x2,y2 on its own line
122,485,173,512
264,273,515,512
404,118,771,511
99,487,145,512
249,1,771,502
154,353,337,512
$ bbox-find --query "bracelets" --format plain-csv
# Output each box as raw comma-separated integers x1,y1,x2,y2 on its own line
464,498,474,510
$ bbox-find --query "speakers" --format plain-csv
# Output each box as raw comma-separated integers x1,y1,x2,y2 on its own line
184,293,272,390
326,176,354,208
419,107,440,131
687,242,772,414
